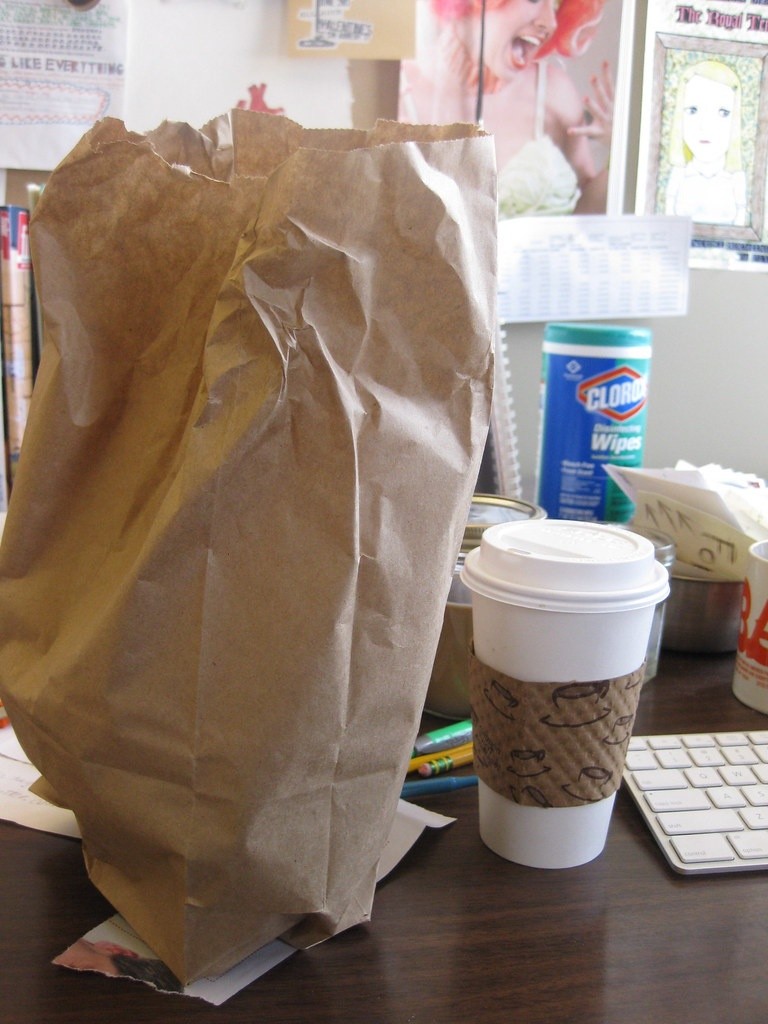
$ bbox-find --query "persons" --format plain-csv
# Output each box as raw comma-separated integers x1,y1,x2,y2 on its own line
400,0,615,213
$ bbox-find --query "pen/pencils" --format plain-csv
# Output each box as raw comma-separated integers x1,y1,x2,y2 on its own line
397,742,479,798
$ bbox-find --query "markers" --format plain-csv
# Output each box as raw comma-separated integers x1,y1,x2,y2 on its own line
411,714,473,760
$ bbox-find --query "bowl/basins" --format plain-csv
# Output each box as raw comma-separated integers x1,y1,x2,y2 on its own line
661,574,743,654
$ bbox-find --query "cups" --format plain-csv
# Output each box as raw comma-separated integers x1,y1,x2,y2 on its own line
621,526,678,685
732,538,768,713
459,519,670,869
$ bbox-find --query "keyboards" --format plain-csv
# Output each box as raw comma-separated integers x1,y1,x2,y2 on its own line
613,730,767,873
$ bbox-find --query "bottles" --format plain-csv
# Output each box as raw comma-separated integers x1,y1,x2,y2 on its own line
535,321,651,526
